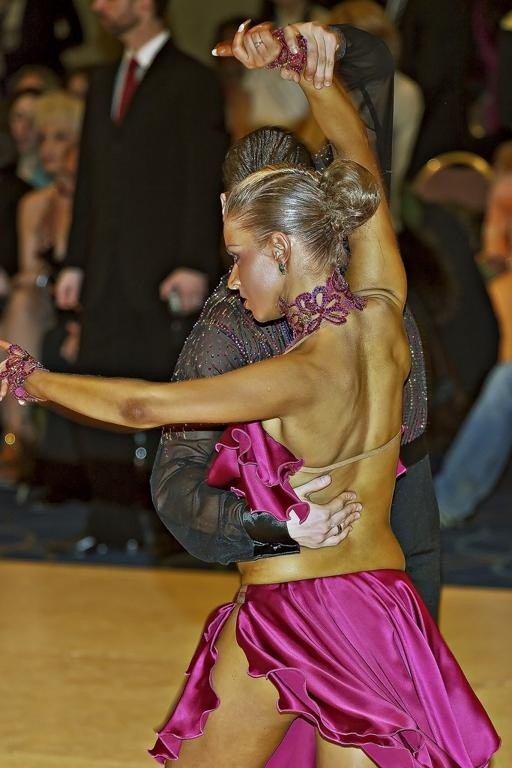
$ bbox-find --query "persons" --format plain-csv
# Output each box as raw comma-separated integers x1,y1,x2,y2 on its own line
149,10,444,630
2,0,512,584
0,17,504,768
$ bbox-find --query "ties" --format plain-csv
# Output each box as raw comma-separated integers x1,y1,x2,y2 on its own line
118,59,139,124
